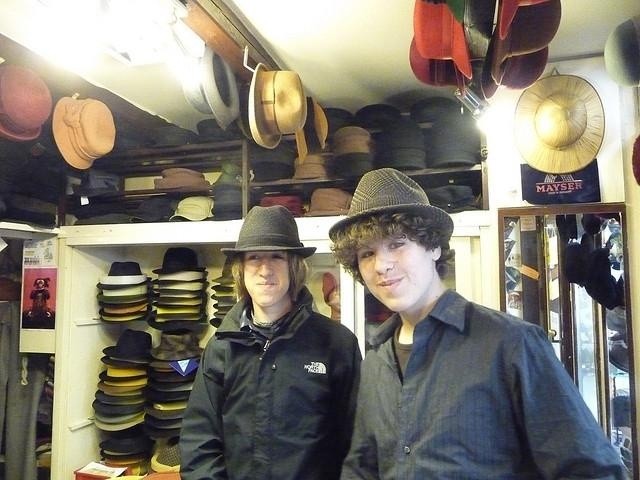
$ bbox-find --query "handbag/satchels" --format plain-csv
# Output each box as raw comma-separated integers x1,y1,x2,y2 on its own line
521,159,600,204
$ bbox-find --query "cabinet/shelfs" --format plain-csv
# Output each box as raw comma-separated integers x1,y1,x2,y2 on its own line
1,117,63,229
61,134,245,228
236,118,491,222
0,224,54,480
352,220,493,359
56,223,356,480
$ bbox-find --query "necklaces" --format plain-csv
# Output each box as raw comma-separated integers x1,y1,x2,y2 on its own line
250,309,279,327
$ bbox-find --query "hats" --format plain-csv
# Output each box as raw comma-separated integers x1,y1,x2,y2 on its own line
130,119,242,223
330,168,454,242
255,97,480,217
410,0,561,100
513,75,605,175
97,248,209,332
92,330,204,473
221,205,317,258
322,273,336,302
184,47,307,148
0,63,127,198
210,256,237,328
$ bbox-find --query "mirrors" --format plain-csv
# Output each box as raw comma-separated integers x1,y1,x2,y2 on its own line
491,199,640,479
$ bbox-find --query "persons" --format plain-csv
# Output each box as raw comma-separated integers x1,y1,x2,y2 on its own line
328,168,629,479
180,205,362,479
